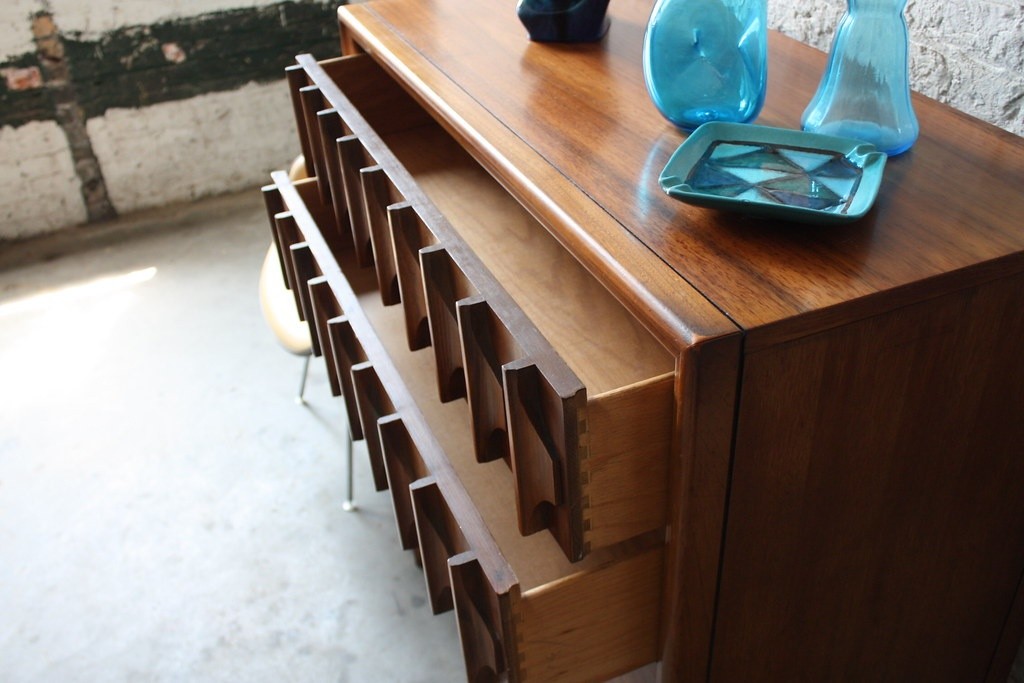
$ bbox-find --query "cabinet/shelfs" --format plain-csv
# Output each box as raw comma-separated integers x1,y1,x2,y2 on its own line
261,0,1023,683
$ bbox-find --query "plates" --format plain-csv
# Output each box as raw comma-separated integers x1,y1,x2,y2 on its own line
659,121,887,224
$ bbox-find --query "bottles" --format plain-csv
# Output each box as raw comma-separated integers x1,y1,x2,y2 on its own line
643,0,768,135
799,0,919,158
